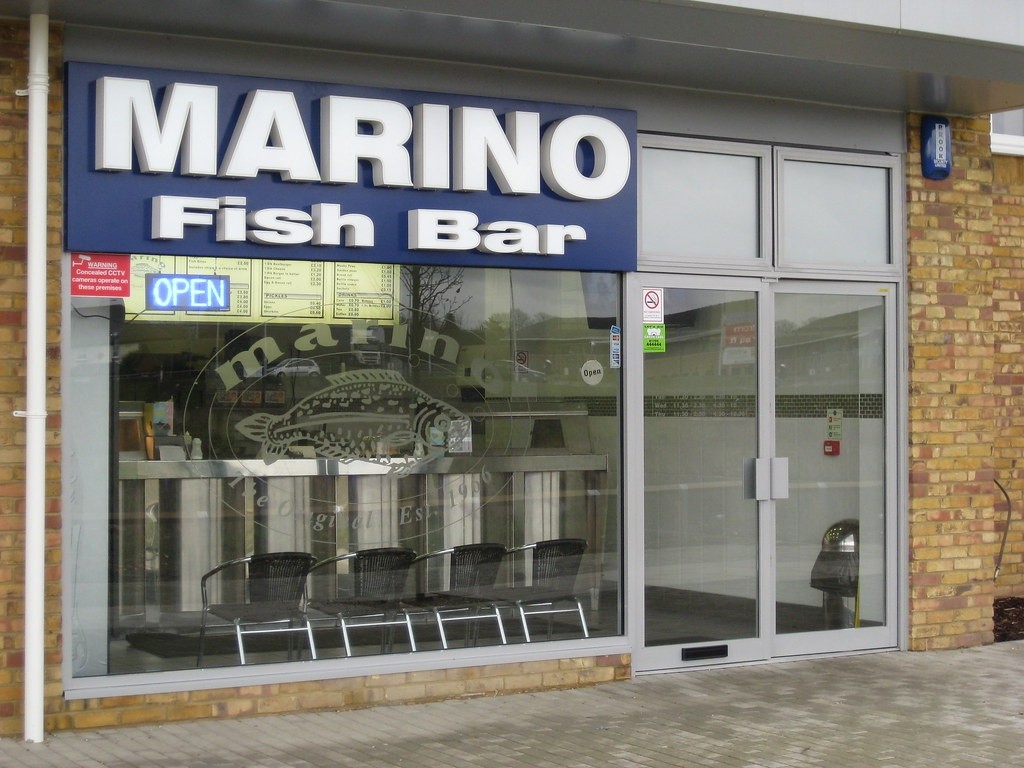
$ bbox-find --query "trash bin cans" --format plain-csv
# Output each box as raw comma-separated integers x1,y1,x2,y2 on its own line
821,518,859,629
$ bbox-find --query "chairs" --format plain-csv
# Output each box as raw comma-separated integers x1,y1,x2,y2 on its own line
471,538,590,647
195,552,317,668
298,547,417,660
389,543,508,654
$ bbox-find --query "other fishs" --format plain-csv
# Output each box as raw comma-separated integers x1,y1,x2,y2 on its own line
233,368,472,477
